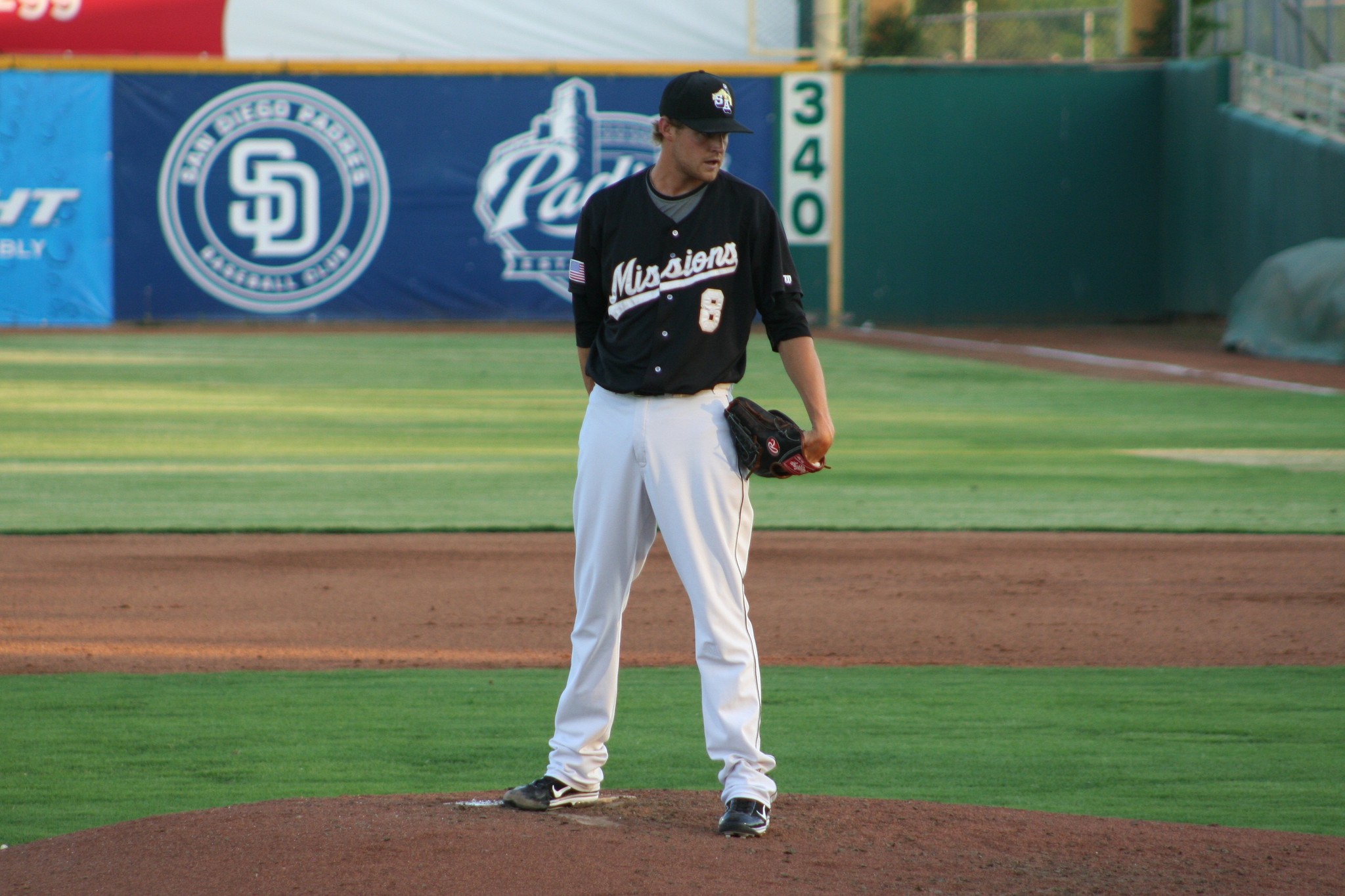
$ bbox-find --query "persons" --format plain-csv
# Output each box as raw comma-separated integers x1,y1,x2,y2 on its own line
502,69,836,842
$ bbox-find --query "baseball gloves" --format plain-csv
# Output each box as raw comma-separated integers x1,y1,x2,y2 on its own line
726,397,832,480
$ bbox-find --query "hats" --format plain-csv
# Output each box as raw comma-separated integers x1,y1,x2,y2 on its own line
660,70,754,134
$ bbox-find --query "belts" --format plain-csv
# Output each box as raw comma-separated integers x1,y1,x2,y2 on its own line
632,388,713,397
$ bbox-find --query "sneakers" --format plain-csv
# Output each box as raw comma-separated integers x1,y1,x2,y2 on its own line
718,797,770,837
501,776,599,811
